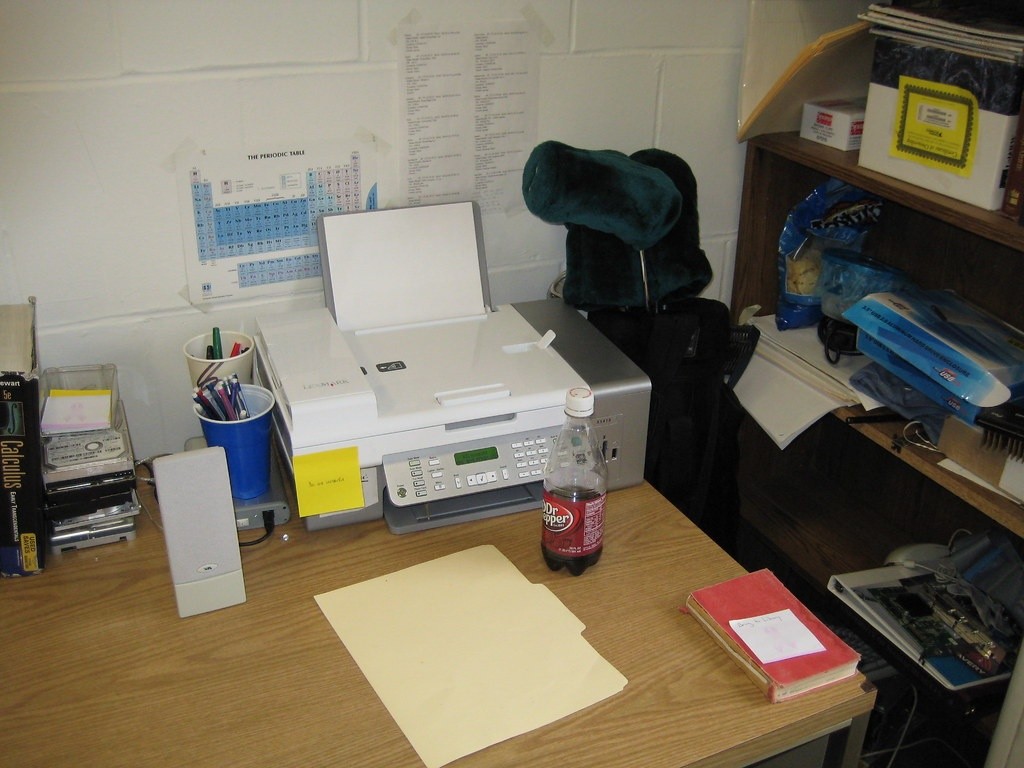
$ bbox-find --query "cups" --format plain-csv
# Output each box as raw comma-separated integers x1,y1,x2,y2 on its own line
193,384,275,498
182,331,255,386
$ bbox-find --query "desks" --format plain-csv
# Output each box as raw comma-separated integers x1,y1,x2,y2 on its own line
0,466,877,768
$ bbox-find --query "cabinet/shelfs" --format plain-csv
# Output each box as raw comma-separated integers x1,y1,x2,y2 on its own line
728,132,1024,767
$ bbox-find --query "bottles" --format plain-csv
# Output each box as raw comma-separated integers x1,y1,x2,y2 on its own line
540,387,608,574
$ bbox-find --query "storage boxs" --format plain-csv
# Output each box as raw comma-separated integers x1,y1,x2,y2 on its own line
857,31,1024,211
799,100,867,153
842,290,1024,424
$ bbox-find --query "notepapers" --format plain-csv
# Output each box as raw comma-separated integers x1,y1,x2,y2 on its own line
41,389,112,434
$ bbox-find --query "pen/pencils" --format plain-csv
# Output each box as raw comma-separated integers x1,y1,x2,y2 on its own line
847,413,906,424
206,327,250,360
192,373,250,421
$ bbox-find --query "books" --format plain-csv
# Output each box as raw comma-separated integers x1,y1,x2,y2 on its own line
1,295,50,578
737,0,1024,217
687,567,862,703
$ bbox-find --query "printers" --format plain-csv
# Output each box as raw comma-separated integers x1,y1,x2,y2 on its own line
249,200,651,530
